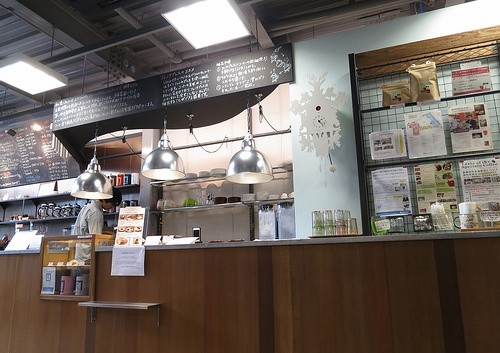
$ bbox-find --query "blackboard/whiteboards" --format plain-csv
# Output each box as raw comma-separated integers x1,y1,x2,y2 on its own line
52,42,294,132
0,119,81,189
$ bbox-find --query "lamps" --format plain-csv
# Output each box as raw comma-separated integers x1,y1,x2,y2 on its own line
159,0,253,51
70,122,114,200
0,54,68,96
226,91,274,185
141,107,186,180
170,51,181,63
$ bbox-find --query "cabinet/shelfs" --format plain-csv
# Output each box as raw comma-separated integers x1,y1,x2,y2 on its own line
148,172,294,213
0,184,139,225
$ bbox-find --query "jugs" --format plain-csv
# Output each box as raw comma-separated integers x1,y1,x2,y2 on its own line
38,204,82,218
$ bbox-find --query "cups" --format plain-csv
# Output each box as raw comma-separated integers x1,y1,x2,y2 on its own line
288,192,295,198
157,188,214,208
108,173,139,186
453,212,479,229
311,210,358,235
103,199,138,213
281,193,287,198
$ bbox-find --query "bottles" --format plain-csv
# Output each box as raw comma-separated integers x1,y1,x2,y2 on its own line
259,206,276,240
277,203,296,240
60,269,73,295
75,268,89,296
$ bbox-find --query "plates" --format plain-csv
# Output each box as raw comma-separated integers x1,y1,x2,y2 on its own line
184,168,226,181
213,192,279,205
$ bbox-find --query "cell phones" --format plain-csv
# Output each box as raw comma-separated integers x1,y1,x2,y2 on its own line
192,227,201,242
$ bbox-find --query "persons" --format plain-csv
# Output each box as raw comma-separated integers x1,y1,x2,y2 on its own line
480,86,484,91
71,186,122,262
449,105,485,133
